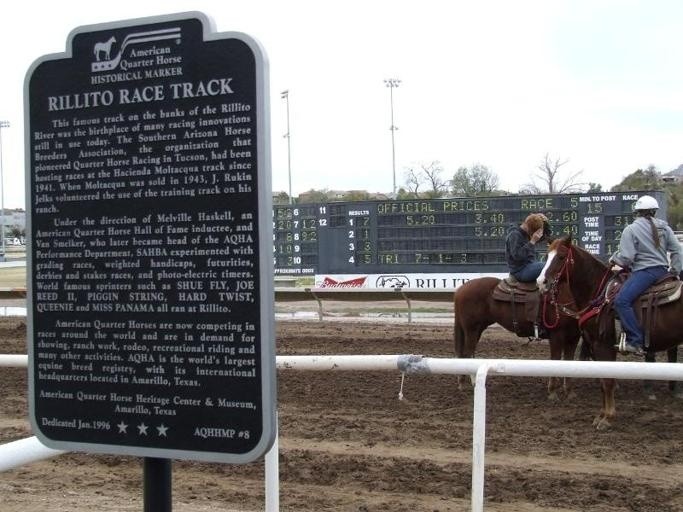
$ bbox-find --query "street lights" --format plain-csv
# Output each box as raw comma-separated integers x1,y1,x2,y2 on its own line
278,87,293,206
0,118,11,254
383,76,402,198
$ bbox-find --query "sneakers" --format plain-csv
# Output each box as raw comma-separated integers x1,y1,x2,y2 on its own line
614,341,643,355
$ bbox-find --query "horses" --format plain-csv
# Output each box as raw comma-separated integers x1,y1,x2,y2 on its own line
535,232,683,431
453,277,580,402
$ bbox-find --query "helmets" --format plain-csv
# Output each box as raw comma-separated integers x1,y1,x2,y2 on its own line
634,194,659,211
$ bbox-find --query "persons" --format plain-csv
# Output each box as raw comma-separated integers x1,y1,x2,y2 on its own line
505,213,550,283
608,196,683,354
